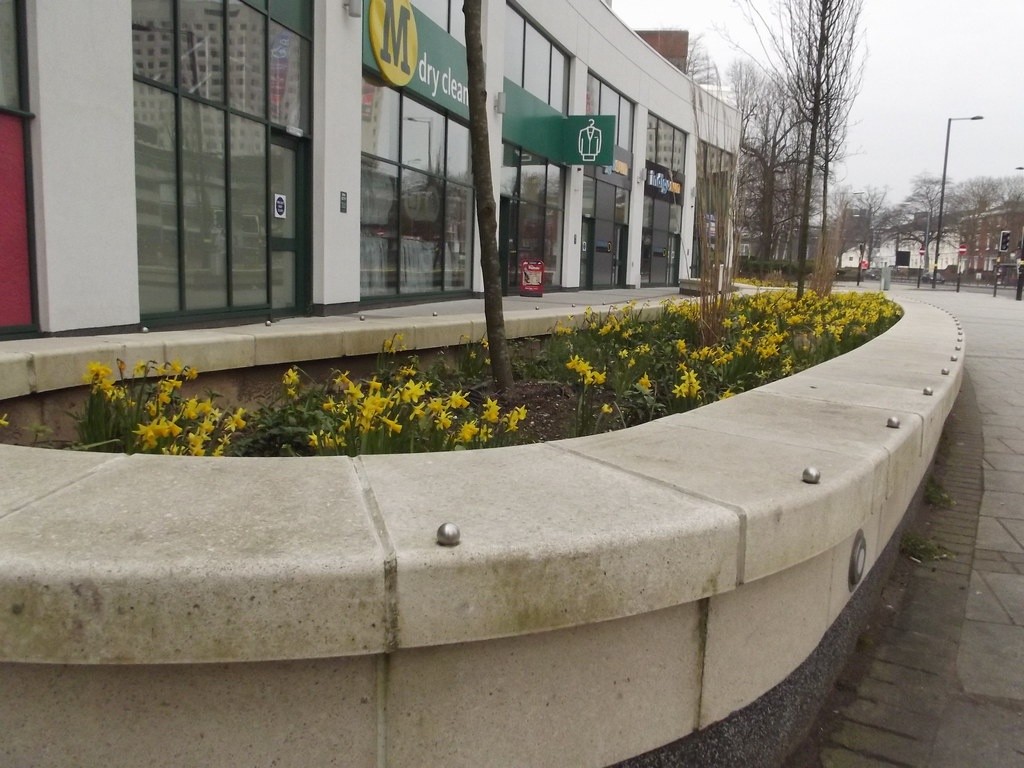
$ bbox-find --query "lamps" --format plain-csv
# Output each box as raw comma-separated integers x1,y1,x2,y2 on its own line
691,186,697,197
494,91,507,114
637,168,647,183
343,0,362,18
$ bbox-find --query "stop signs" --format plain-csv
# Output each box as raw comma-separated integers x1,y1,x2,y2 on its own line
918,248,925,255
958,244,968,256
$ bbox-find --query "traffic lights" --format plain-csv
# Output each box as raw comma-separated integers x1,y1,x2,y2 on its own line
999,230,1011,252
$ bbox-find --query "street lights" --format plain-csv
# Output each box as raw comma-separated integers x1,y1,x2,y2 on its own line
131,22,213,272
904,202,931,273
932,115,986,288
408,117,433,186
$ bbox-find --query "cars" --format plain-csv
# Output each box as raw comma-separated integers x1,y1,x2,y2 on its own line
866,267,882,280
922,272,945,286
